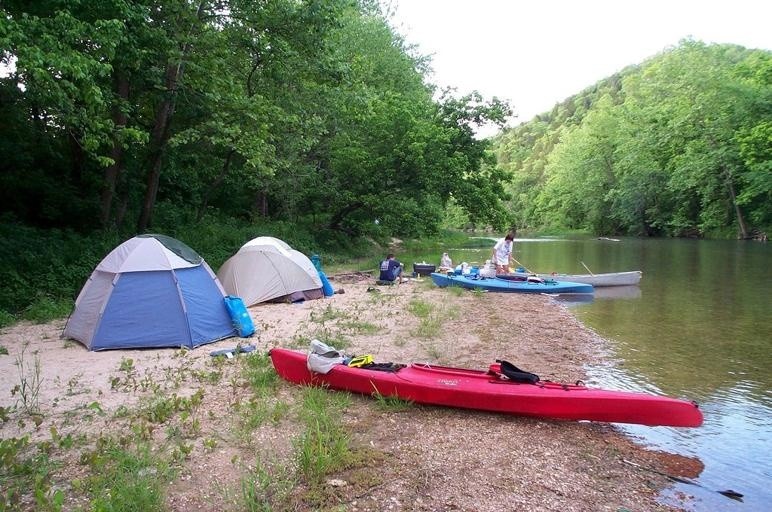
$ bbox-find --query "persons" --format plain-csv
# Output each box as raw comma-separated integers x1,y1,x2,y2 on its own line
379,254,404,284
492,233,514,275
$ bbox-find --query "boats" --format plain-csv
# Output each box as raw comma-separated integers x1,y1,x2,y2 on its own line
268,348,702,427
430,267,643,294
597,238,620,241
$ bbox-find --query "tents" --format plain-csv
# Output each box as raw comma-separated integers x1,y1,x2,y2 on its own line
62,234,255,350
217,236,325,307
